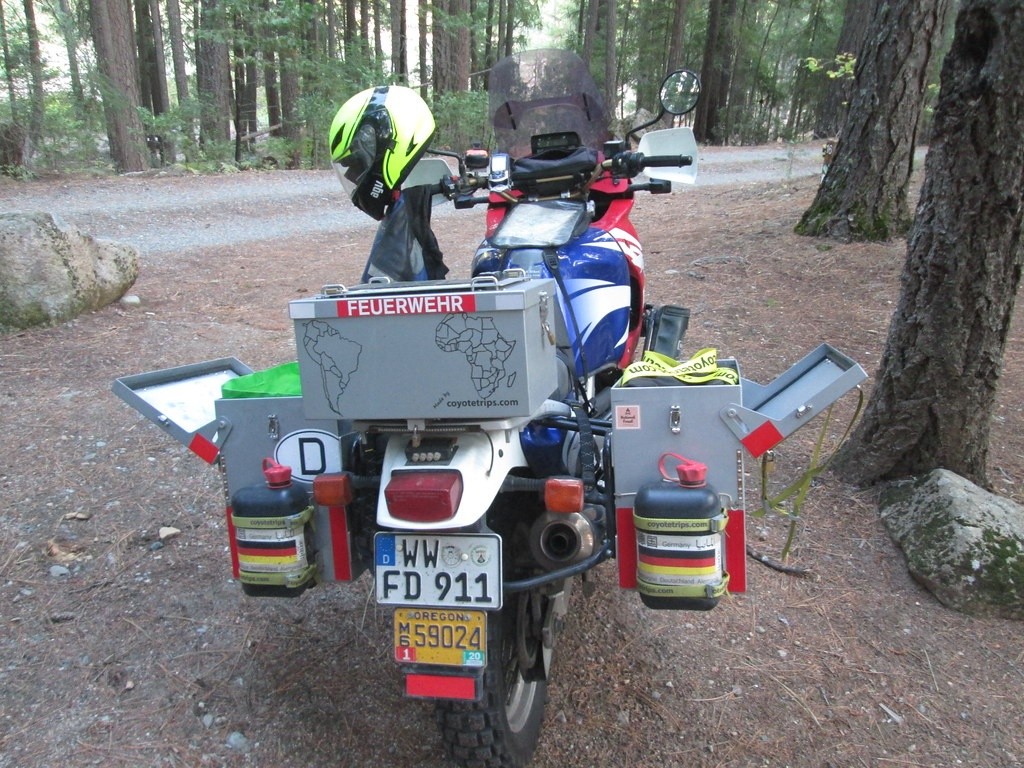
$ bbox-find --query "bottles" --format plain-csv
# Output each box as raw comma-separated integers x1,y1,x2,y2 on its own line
633,452,730,612
231,456,315,598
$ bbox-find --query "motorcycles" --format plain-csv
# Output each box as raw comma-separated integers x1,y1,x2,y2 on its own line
110,48,871,768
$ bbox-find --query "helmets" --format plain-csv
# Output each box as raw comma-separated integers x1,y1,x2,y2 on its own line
330,86,435,222
